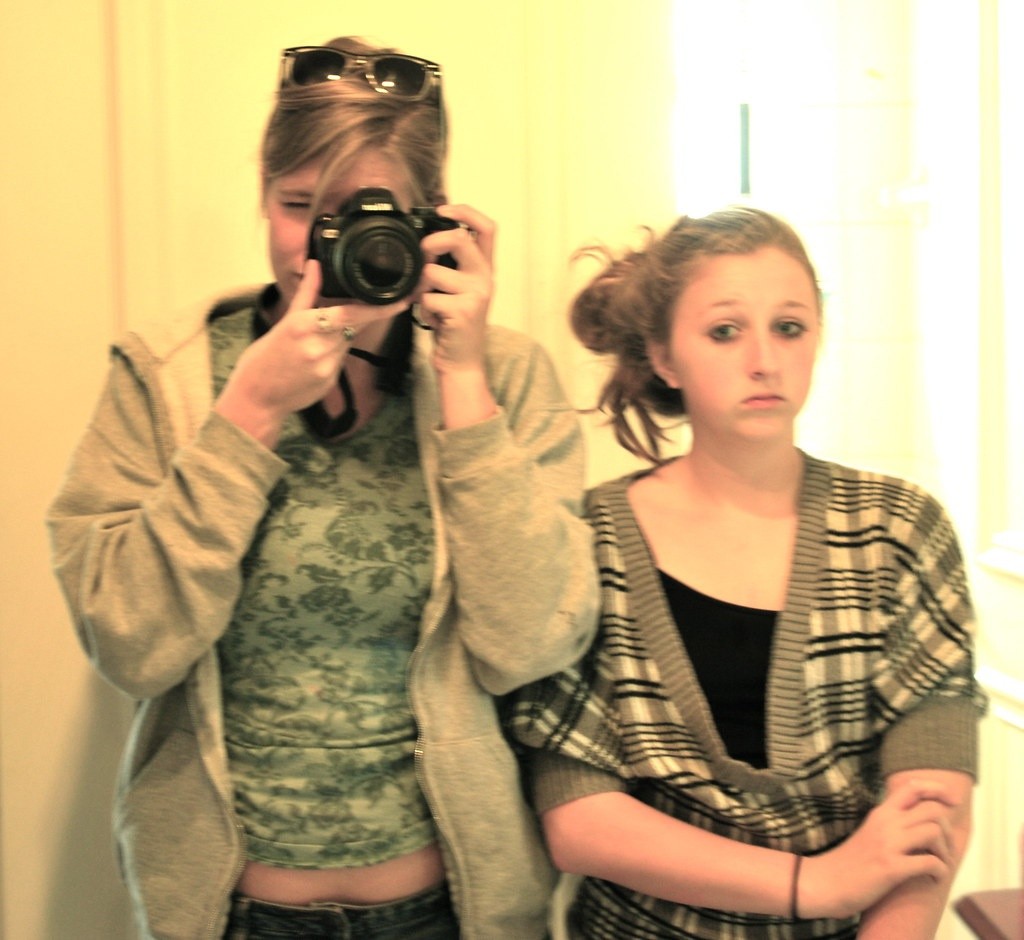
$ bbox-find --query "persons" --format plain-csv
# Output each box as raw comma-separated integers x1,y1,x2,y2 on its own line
45,36,601,940
503,207,990,940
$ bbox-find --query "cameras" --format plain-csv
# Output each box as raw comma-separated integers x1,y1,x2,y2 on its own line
306,186,460,306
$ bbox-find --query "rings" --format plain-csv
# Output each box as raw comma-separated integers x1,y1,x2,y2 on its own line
317,307,330,330
343,328,356,340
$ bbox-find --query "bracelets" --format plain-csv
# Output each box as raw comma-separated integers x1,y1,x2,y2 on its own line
791,854,802,920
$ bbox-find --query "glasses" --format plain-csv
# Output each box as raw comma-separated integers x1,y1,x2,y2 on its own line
280,46,446,165
